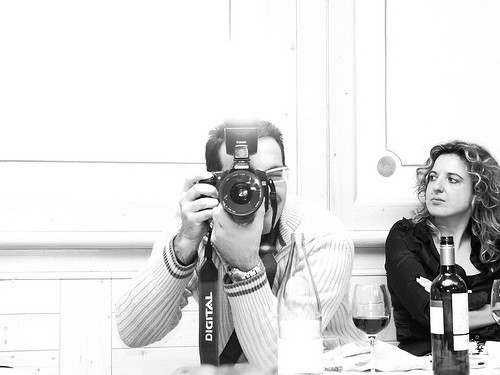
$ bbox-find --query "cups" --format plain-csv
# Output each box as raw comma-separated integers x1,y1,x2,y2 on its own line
322,336,343,375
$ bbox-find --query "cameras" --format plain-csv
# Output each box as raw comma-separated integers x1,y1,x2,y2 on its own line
207,127,270,226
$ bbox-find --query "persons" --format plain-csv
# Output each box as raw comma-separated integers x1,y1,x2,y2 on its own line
384,141,499,341
114,120,368,365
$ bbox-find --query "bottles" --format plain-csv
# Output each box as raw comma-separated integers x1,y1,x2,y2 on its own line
430,235,470,375
278,232,323,375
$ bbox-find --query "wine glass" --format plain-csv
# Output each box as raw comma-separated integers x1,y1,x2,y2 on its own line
491,279,500,369
352,284,391,375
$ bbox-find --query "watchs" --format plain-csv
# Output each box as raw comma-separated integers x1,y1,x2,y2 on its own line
226,258,264,283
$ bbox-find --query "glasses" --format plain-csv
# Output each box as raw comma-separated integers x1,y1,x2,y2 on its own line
266,166,288,182
